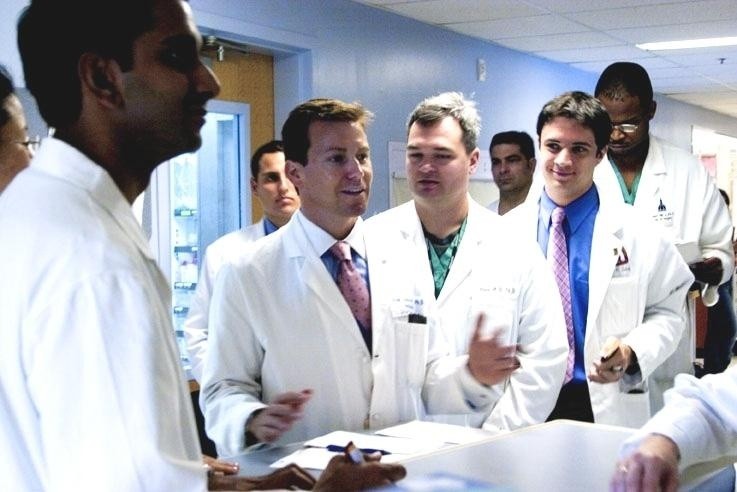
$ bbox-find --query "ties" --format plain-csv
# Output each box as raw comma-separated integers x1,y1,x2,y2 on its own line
545,206,575,386
329,240,372,339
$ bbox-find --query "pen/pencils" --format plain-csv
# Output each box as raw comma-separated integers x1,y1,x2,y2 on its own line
326,445,392,455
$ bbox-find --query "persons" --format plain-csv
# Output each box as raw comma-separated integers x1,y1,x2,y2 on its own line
595,61,736,491
487,131,540,216
364,93,568,433
1,0,404,492
500,91,695,428
183,139,302,388
198,99,523,456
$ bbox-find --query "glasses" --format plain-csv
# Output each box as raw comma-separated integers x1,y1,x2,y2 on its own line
611,113,650,135
2,133,41,154
